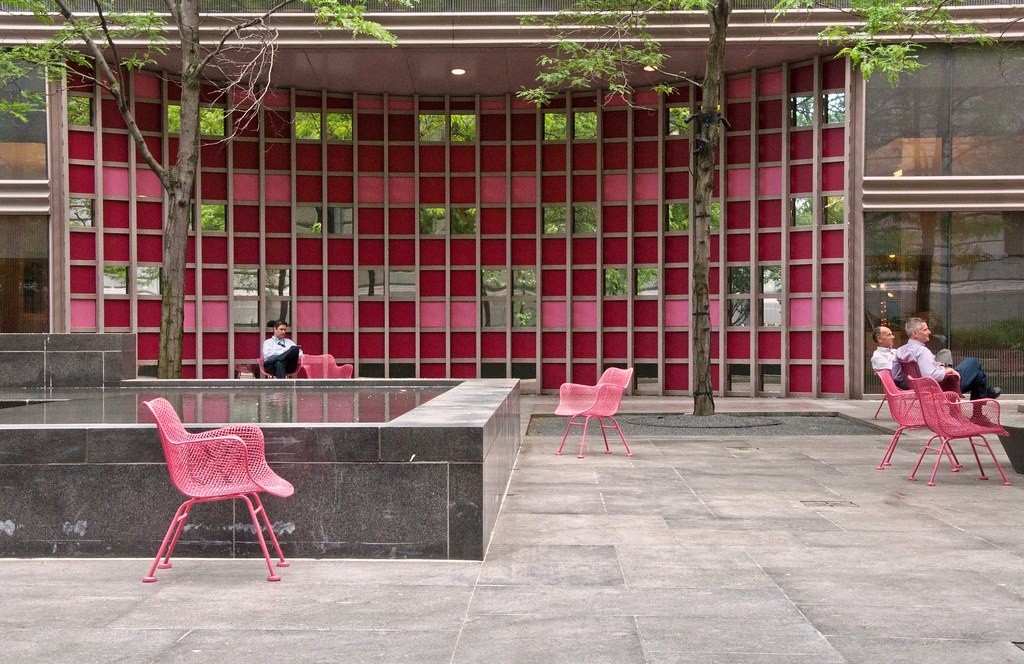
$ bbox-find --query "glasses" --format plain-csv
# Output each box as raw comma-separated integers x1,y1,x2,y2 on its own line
940,363,953,367
278,342,285,347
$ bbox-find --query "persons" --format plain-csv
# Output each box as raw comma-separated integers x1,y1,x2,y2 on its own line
871,326,953,371
892,318,1002,424
262,320,303,378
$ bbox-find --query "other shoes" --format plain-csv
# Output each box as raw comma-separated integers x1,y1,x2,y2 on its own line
264,357,275,370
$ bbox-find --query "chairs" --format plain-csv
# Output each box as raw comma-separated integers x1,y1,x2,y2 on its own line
874,357,964,471
552,367,634,458
907,374,1011,488
141,397,295,582
257,353,305,379
298,353,353,379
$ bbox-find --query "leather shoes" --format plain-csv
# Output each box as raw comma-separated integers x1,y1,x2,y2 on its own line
985,387,1001,399
971,415,992,426
